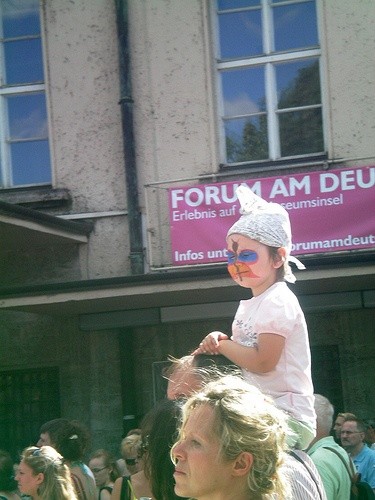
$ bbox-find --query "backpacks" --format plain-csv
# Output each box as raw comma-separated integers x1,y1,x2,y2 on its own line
323,447,375,500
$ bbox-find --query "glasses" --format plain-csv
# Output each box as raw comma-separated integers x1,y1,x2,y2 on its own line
91,466,108,474
126,456,142,464
340,430,362,435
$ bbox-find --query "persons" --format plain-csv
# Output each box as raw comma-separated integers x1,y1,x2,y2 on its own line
192,203,317,452
0,353,375,500
